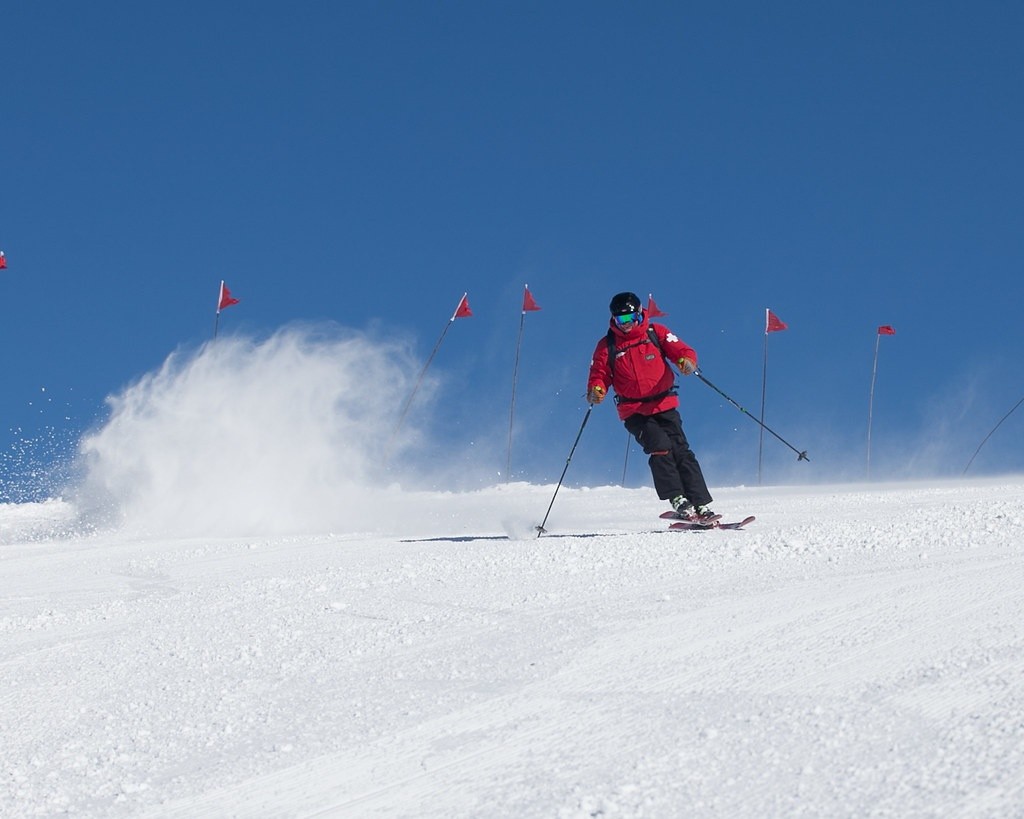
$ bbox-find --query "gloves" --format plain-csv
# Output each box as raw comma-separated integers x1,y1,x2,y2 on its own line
586,386,606,404
678,357,697,376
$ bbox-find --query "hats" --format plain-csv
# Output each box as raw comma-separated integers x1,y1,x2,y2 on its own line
609,292,641,316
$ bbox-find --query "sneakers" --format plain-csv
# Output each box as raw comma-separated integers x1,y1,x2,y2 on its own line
670,494,694,514
696,505,715,519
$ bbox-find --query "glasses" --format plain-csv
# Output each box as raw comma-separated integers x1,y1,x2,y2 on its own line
615,312,639,324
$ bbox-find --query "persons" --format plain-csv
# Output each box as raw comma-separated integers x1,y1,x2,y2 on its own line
586,292,715,517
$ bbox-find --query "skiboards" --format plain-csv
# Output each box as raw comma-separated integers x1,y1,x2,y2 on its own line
658,510,757,531
